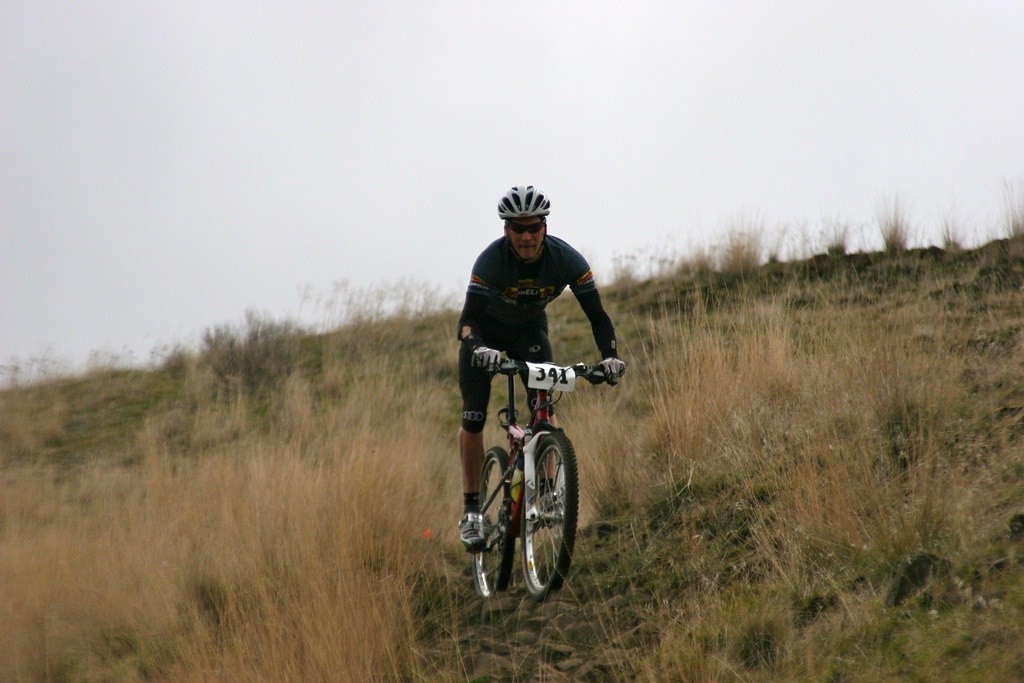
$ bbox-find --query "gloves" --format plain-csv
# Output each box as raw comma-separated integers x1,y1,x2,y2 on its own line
470,346,501,375
598,356,626,386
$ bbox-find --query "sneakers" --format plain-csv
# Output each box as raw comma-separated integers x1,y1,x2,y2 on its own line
539,492,563,529
458,512,486,551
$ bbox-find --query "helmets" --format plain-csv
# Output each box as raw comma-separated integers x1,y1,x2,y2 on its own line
497,184,551,222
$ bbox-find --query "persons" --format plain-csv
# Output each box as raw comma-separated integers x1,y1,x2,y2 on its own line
457,186,628,547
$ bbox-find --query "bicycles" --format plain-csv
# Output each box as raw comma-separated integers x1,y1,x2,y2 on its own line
472,351,618,604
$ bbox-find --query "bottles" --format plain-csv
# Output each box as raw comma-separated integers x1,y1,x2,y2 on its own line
510,453,525,503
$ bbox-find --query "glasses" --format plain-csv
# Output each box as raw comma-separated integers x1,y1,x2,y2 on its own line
508,217,546,234
